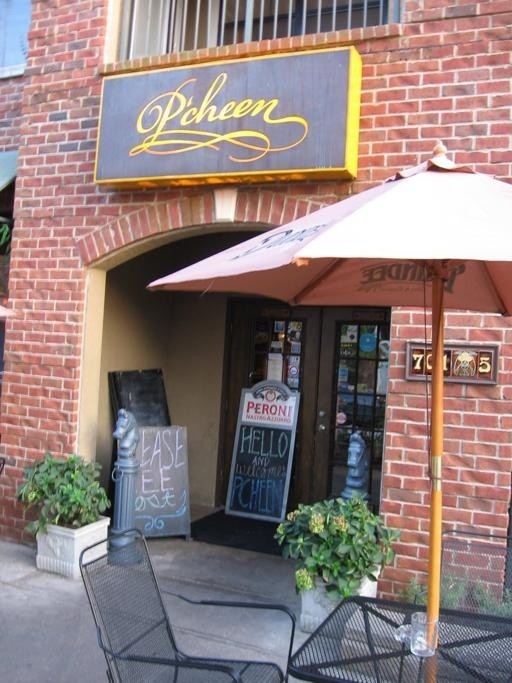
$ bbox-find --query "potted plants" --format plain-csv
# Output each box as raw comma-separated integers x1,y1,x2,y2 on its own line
10,448,113,584
272,491,403,642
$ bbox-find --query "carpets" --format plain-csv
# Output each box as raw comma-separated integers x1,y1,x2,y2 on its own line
180,507,292,559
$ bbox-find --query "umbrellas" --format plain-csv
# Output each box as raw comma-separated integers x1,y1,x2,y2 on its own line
146,131,512,679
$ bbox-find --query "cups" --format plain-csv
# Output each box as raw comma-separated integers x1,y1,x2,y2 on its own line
410,611,439,658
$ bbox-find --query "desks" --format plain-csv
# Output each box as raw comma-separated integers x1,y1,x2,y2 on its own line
287,594,512,683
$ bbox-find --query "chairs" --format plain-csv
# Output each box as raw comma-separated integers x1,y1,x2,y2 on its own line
79,529,298,683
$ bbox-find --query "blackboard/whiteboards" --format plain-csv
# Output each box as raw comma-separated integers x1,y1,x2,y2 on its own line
225,380,301,523
115,367,190,536
